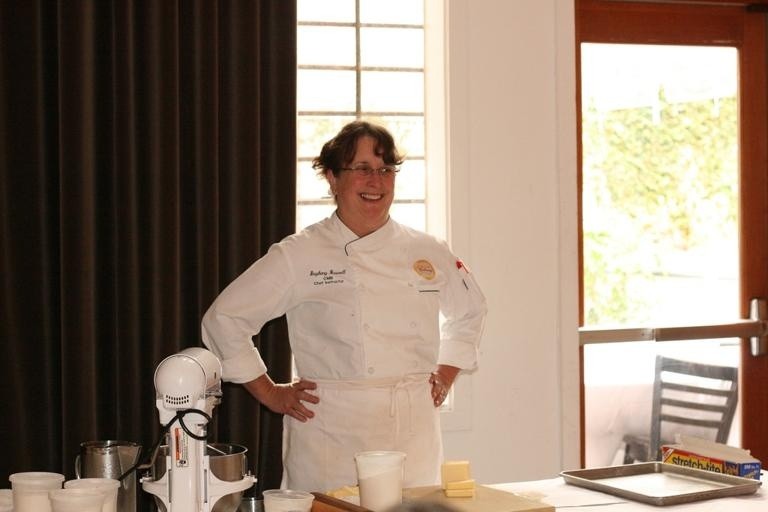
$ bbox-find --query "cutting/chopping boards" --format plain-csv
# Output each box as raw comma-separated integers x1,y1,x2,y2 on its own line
310,480,557,511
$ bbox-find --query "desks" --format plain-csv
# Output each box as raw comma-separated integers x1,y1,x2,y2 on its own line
252,462,768,512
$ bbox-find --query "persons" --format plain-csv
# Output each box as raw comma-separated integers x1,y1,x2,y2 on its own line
199,120,489,497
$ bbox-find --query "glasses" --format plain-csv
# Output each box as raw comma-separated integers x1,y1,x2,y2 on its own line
339,165,400,176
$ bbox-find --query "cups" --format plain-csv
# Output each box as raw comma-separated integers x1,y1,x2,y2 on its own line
9,471,64,511
50,488,107,512
354,450,407,512
63,477,121,511
262,489,314,511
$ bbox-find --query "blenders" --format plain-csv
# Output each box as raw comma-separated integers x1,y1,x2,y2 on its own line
138,347,259,512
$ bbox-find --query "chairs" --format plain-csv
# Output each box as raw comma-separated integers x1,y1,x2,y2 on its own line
620,353,741,469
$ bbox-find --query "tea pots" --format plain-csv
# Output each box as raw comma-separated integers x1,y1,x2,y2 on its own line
75,440,142,512
